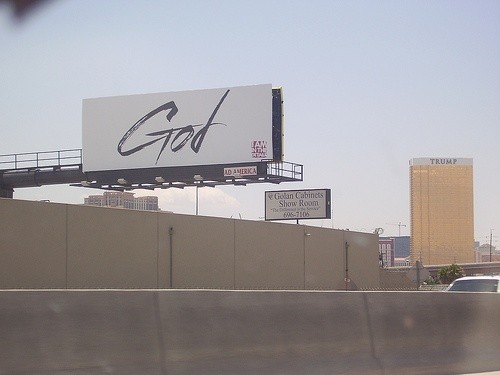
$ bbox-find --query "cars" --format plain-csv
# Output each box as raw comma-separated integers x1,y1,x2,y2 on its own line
445,276,500,293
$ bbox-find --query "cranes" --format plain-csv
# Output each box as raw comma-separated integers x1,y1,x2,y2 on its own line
387,221,407,237
